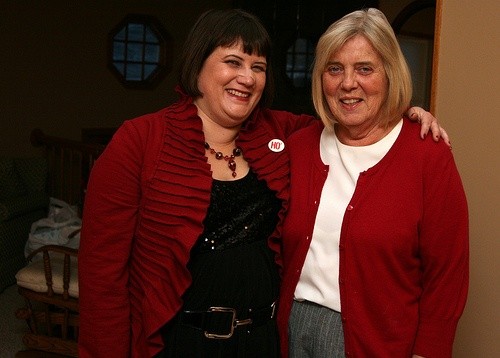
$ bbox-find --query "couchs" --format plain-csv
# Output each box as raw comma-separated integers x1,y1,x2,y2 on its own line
0,153,72,291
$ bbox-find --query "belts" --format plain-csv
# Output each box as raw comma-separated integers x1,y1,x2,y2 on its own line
169,298,278,339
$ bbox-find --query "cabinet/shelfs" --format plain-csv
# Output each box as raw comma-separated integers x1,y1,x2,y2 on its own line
80,127,120,175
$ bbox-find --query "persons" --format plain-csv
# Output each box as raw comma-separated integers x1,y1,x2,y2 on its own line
278,8,473,357
78,10,453,358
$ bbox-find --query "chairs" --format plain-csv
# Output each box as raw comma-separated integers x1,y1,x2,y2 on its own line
12,127,106,358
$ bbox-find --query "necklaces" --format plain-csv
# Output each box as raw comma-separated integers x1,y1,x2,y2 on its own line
204,141,242,179
